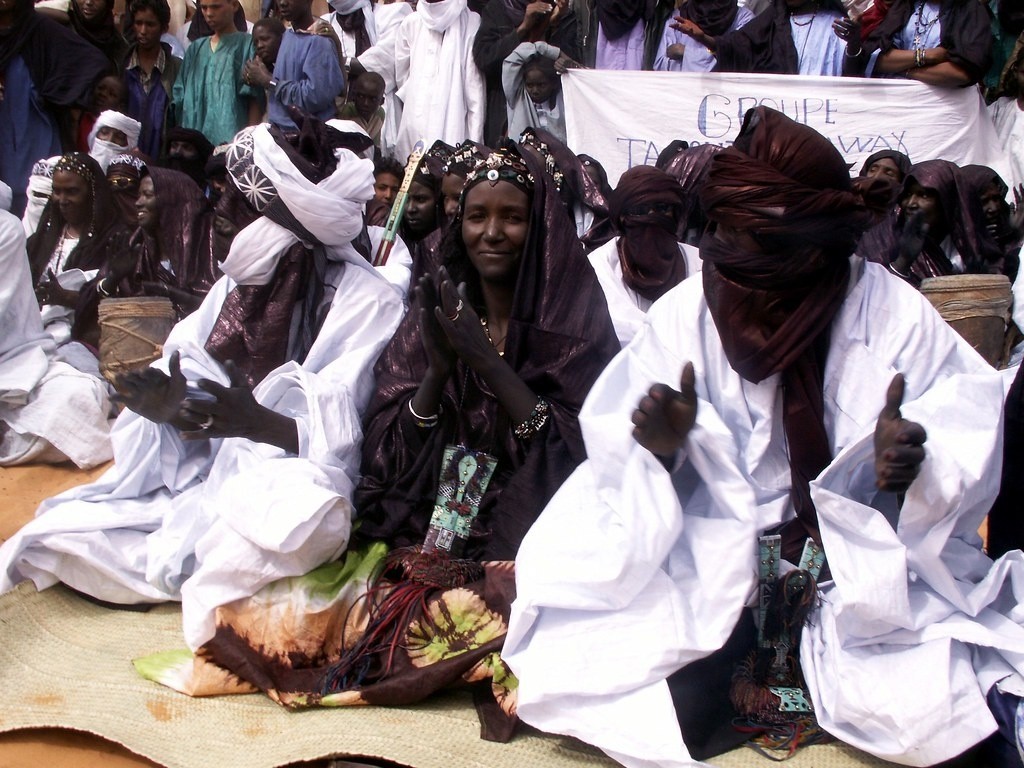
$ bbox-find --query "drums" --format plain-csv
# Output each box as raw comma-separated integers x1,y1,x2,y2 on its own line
97,294,177,416
922,272,1013,371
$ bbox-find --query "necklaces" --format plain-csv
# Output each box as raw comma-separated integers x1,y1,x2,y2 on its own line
480,316,506,348
421,364,498,554
750,420,825,686
915,0,939,34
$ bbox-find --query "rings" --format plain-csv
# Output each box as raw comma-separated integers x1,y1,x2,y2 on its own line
199,416,213,429
46,295,49,300
845,32,849,36
326,28,328,31
448,310,459,321
456,299,464,311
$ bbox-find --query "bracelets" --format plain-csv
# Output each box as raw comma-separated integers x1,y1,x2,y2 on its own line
268,78,278,91
845,45,862,58
96,278,120,299
163,285,168,296
408,398,443,427
513,395,551,438
915,48,926,66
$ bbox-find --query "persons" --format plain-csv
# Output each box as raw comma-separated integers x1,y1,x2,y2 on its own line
0,104,407,654
0,208,114,471
504,104,1024,768
0,0,1024,416
131,131,621,746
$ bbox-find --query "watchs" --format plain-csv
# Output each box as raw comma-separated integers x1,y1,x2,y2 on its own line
345,57,352,73
